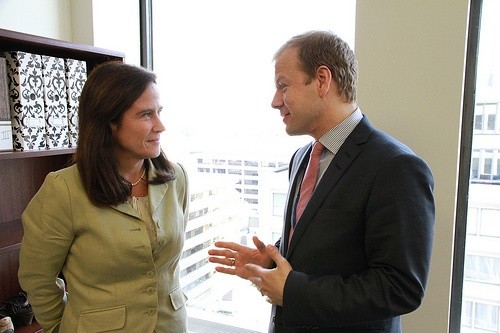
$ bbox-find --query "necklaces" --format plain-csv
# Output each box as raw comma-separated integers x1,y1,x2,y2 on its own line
120,168,146,187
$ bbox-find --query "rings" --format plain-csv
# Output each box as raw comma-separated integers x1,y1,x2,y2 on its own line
231,260,235,266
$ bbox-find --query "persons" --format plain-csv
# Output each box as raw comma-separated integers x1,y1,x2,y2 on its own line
208,31,436,333
17,62,192,333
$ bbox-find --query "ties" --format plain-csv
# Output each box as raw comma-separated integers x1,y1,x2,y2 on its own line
288,140,326,250
